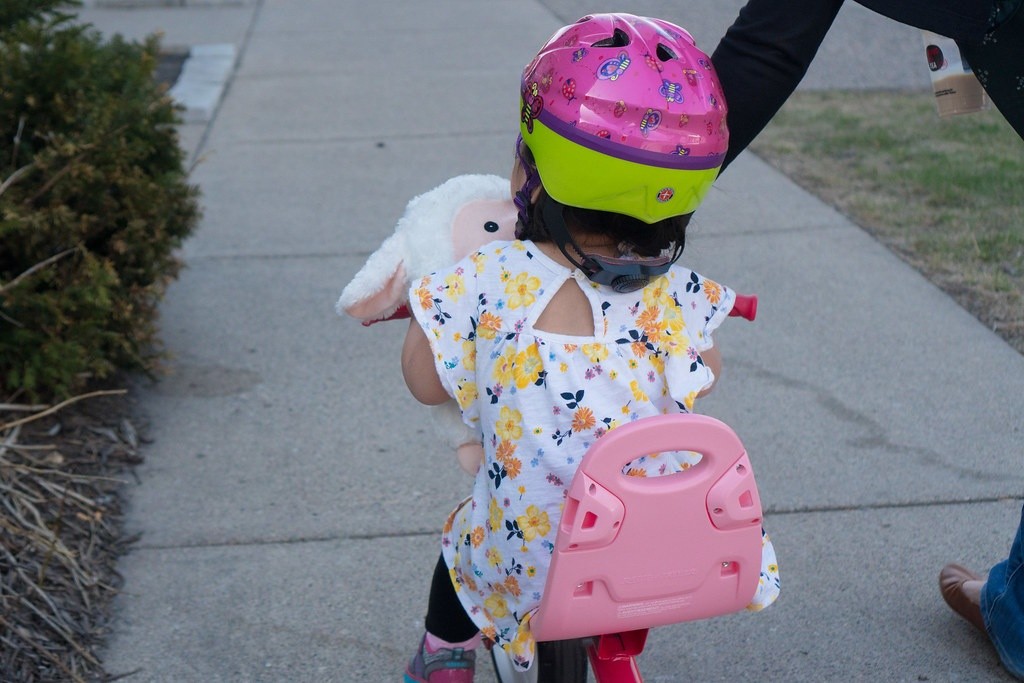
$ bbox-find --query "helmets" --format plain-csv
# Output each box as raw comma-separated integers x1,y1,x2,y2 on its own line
516,11,729,223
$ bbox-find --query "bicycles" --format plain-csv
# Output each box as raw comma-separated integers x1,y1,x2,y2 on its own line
357,284,759,683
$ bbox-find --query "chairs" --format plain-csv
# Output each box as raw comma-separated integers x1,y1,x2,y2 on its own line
522,415,762,640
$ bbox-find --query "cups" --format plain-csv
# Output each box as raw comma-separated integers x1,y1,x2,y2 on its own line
921,28,991,118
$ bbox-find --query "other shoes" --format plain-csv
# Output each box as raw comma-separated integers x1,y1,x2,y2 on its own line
940,563,991,641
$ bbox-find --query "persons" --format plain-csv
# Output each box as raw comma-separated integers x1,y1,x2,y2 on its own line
710,0,1024,683
404,13,738,683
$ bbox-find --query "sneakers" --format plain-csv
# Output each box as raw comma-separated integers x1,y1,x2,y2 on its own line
403,633,477,683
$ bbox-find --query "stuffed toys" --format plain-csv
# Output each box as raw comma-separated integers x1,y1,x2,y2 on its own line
337,171,755,322
